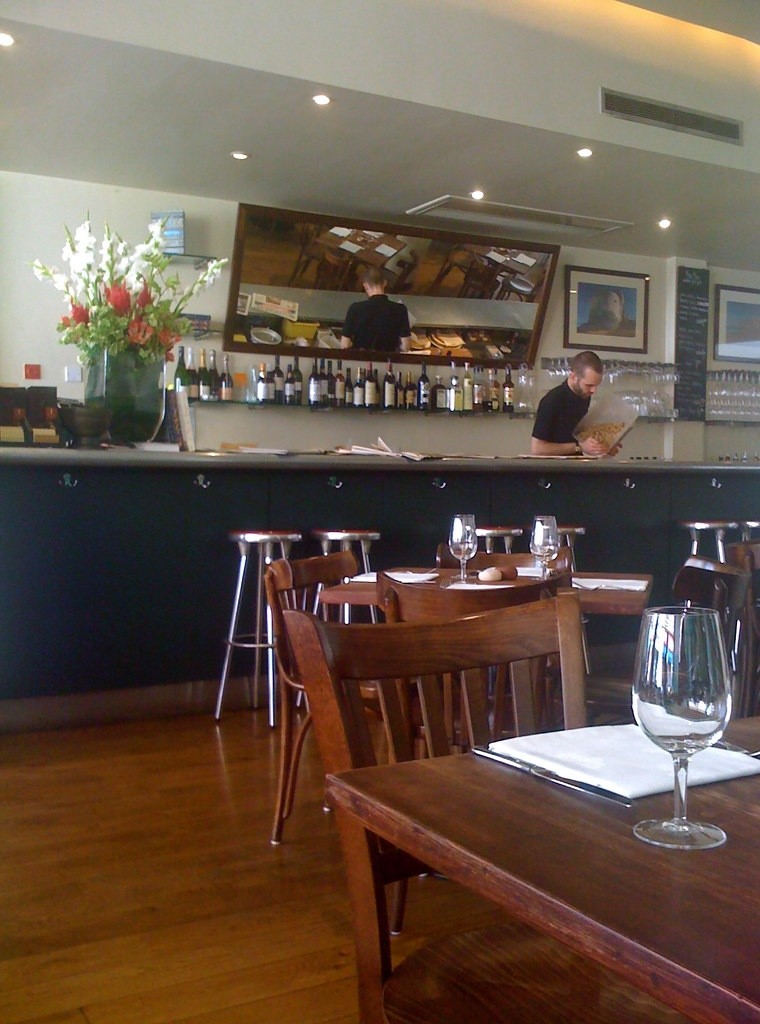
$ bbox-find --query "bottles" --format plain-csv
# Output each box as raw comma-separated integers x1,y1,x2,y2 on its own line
247,354,303,405
173,345,234,402
308,357,534,413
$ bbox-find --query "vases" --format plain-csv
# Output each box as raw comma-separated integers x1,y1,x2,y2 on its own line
86,346,166,443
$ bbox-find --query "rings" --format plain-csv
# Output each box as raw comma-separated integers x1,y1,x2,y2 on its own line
596,451,598,454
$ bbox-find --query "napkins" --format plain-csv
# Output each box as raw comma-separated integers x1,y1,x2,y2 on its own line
485,251,506,263
572,577,649,592
351,572,440,584
515,567,554,577
513,253,536,266
490,724,760,799
444,583,515,589
330,226,397,257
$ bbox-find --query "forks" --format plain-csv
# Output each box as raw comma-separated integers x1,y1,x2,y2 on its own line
717,740,760,756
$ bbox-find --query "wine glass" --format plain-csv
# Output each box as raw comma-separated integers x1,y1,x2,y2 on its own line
530,515,560,580
631,605,733,850
621,389,679,417
546,358,680,384
449,514,478,585
707,370,760,421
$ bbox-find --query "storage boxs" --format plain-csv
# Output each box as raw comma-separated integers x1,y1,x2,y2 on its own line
282,318,320,339
151,212,185,254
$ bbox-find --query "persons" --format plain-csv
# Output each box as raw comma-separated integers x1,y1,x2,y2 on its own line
339,267,412,353
531,351,623,457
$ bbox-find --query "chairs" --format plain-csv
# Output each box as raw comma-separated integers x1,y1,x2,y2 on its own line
291,220,350,291
428,241,549,304
264,539,760,1024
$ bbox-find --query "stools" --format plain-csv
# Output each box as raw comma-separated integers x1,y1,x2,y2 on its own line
475,523,592,693
679,519,760,672
215,528,380,725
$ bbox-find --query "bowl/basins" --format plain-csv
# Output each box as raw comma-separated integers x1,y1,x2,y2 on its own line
59,404,113,438
251,326,282,346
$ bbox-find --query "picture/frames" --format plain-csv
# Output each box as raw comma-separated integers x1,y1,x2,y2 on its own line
565,265,649,354
713,284,760,364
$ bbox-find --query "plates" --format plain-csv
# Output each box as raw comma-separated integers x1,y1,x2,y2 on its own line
412,327,463,349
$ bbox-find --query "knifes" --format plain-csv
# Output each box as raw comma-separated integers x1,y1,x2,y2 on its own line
472,747,636,808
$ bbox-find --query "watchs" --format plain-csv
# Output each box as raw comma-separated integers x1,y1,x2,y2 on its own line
575,442,581,455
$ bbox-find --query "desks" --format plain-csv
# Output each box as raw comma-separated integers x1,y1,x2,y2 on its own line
315,227,407,292
324,714,760,1024
462,243,537,299
318,567,653,758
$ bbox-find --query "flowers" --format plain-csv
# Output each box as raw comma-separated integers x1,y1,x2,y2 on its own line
33,209,229,363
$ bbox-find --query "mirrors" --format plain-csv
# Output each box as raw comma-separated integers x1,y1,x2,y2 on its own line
222,202,561,370
